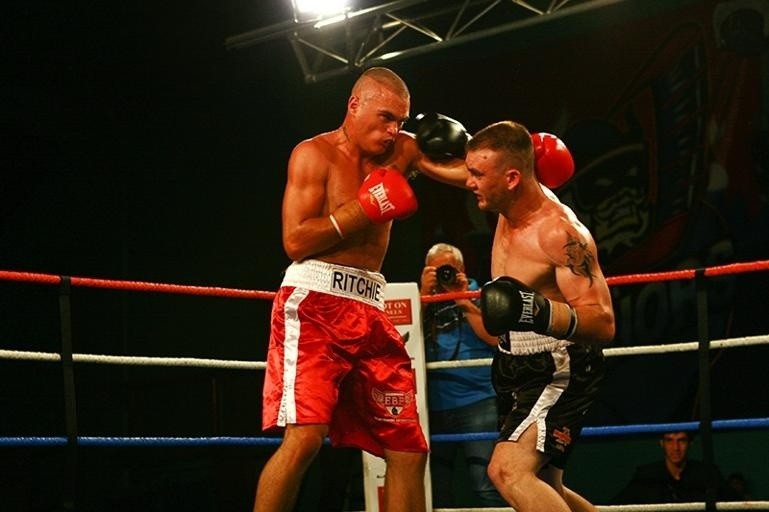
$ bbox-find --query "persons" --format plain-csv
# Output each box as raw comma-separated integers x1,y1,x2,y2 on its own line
610,429,712,501
723,472,757,501
252,68,575,512
414,110,616,512
418,242,508,508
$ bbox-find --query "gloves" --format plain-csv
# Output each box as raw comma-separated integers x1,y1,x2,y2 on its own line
413,110,474,165
329,164,419,245
528,131,575,190
479,274,580,343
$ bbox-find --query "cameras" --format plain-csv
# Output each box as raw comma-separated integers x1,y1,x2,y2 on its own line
436,265,457,285
